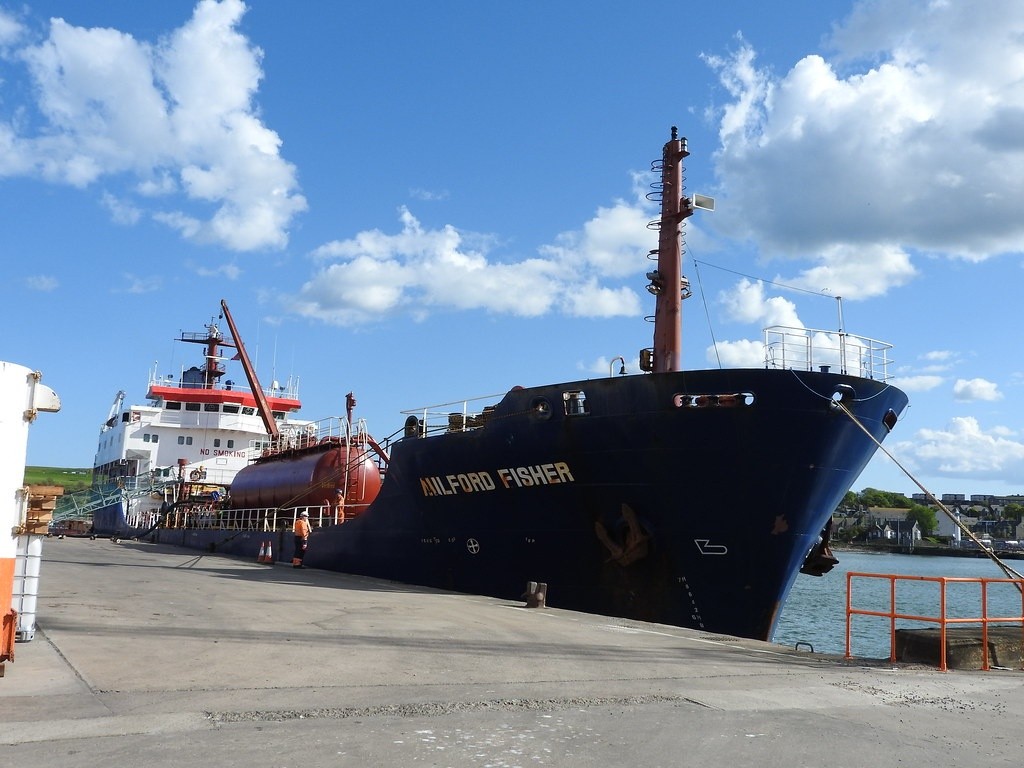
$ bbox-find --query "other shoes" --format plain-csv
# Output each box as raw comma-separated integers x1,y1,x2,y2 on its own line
293,565,307,569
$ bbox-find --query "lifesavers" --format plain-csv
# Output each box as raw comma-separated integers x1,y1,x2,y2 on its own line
190,470,202,482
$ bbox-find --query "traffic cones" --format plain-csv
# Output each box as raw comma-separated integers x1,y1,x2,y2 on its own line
257,541,265,564
262,541,273,564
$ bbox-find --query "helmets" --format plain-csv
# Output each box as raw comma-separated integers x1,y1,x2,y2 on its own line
334,489,342,495
300,511,309,517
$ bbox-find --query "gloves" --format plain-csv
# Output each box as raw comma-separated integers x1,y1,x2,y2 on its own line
302,544,307,549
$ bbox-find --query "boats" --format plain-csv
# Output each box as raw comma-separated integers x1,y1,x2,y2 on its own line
92,124,911,643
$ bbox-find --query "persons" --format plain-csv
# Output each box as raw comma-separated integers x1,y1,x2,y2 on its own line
319,496,331,528
330,489,345,526
292,511,309,570
